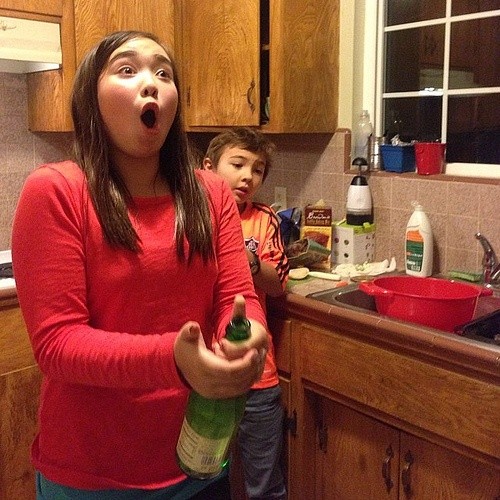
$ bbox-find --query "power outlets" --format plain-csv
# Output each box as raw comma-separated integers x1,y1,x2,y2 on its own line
273,186,286,206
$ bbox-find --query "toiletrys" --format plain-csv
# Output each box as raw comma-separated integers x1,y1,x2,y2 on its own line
346,157,374,226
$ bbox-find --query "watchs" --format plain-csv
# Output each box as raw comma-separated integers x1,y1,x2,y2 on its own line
249,251,261,275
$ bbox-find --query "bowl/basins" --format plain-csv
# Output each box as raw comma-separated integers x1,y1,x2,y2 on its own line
414,143,446,174
381,144,416,173
359,274,493,331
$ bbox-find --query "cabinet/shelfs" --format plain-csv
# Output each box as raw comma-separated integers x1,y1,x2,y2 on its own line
0,267,500,500
0,0,338,134
419,1,500,87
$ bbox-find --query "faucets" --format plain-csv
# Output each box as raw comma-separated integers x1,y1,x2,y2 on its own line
474,233,499,285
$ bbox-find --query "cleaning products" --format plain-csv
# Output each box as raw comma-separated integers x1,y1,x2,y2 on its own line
404,199,434,278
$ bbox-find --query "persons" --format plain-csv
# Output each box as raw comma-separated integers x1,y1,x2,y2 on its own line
201,126,290,500
10,29,273,500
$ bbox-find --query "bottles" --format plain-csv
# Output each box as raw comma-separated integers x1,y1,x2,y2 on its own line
351,110,372,171
404,200,432,278
175,315,251,479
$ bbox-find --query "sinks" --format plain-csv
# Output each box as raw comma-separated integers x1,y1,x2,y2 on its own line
305,270,500,352
